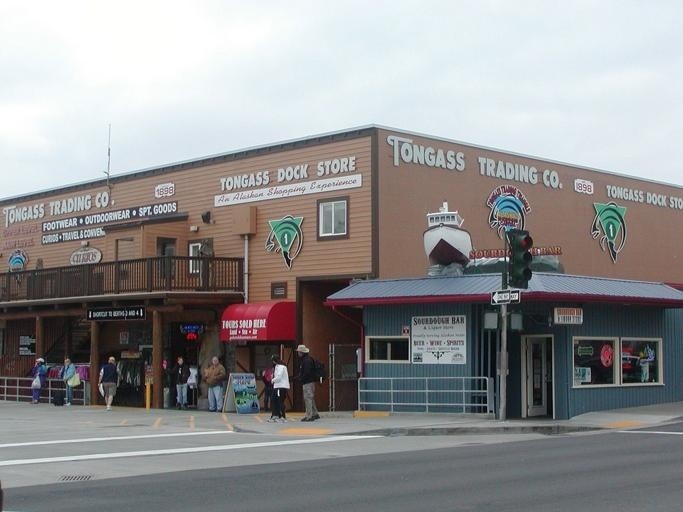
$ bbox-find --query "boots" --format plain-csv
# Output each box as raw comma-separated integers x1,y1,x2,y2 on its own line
176,403,188,410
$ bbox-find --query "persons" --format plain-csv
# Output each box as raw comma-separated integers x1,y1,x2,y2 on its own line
98,356,122,411
31,358,47,404
262,354,290,422
60,358,76,406
290,345,320,421
205,356,226,412
171,356,191,410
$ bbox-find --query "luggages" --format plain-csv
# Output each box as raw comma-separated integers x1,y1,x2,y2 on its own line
53,378,63,406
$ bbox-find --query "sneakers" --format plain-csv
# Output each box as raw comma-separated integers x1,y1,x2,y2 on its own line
267,416,288,423
301,414,320,421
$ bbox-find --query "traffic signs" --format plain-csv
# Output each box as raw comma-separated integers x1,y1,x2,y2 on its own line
488,288,522,305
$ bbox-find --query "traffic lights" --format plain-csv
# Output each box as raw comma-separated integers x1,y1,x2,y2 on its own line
508,227,536,289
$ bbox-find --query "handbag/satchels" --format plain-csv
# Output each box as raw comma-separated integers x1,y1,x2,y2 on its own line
67,373,81,387
32,377,41,390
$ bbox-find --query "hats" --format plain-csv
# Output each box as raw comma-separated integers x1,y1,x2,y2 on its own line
295,344,309,353
36,358,45,363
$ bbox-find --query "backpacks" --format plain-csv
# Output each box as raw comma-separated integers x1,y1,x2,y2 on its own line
310,355,325,382
